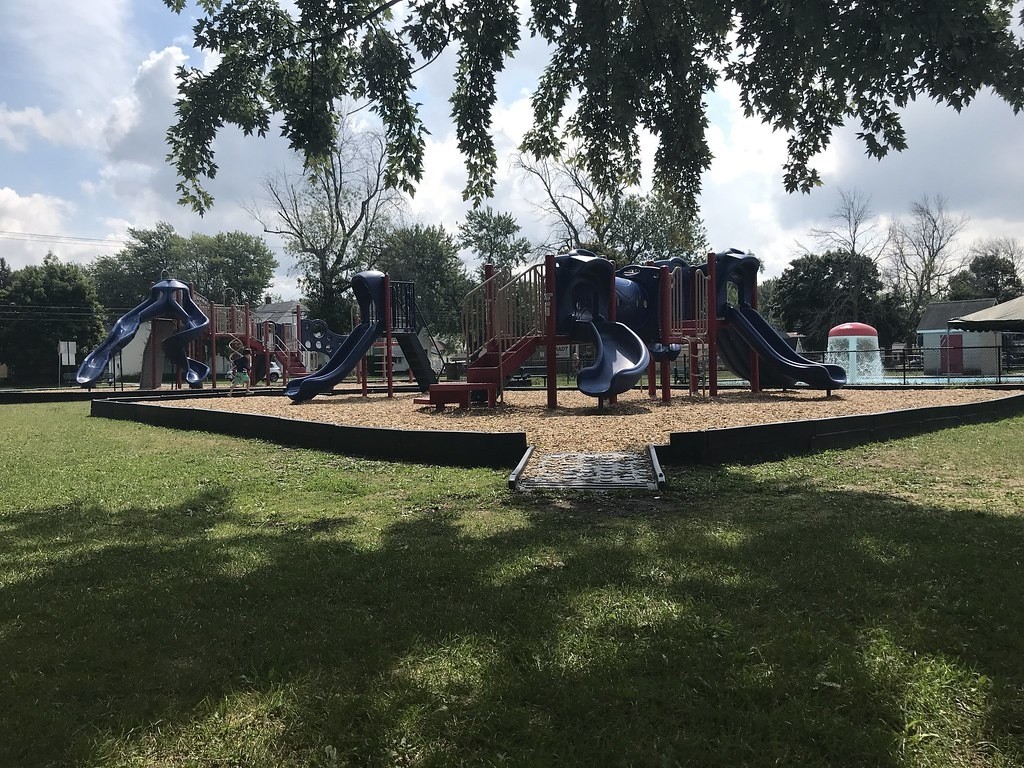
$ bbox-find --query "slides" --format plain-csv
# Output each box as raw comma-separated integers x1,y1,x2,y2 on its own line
716,304,847,390
161,297,210,388
75,297,163,385
284,322,382,402
574,320,650,398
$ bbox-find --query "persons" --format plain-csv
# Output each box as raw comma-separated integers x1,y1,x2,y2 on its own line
227,348,254,397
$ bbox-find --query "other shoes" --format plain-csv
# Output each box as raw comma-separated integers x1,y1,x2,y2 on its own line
245,391,255,396
227,394,237,397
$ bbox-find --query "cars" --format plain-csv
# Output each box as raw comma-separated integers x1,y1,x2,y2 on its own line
225,362,282,382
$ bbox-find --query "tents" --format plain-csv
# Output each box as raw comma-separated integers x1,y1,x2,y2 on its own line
947,295,1024,383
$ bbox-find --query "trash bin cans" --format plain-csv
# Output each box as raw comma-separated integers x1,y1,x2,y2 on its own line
443,362,462,380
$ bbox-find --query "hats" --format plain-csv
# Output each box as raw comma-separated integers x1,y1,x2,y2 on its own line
241,349,254,355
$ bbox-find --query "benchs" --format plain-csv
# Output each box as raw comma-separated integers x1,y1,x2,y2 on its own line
63,373,113,386
513,367,547,387
674,367,708,383
1002,357,1024,371
